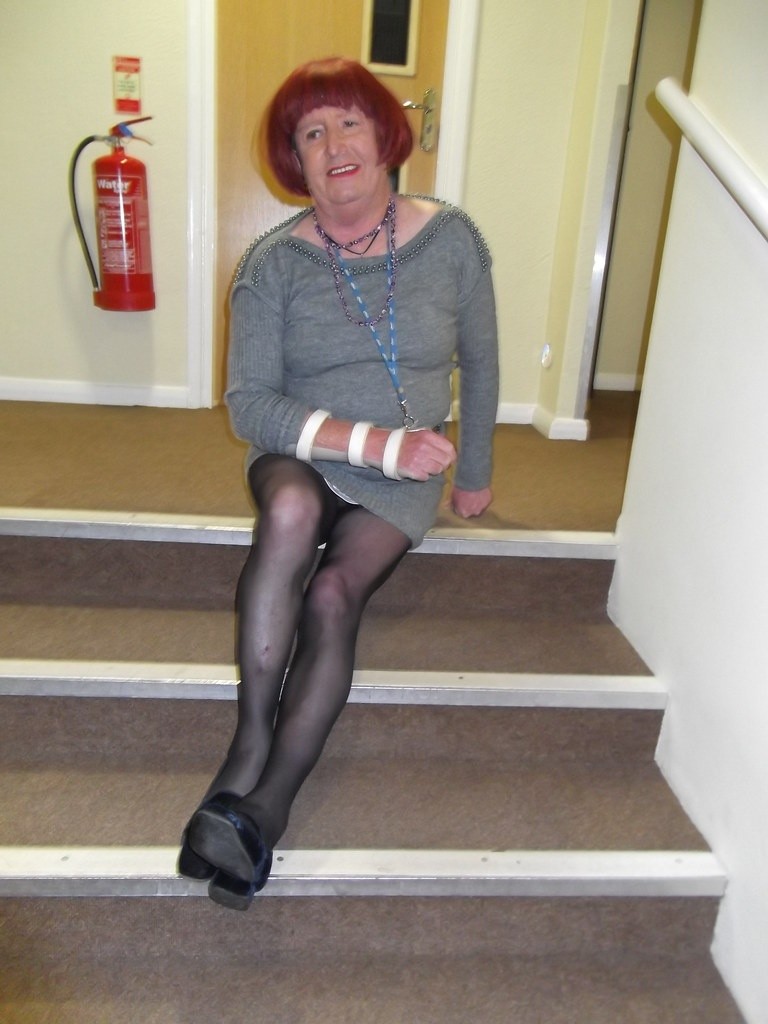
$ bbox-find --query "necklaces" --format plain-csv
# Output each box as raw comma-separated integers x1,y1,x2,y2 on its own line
305,195,399,329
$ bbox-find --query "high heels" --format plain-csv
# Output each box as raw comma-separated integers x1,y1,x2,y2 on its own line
187,803,272,911
179,788,243,879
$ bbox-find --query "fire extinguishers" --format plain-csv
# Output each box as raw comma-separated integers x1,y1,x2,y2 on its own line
69,113,156,313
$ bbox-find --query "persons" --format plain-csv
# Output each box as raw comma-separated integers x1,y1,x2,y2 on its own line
178,56,501,910
